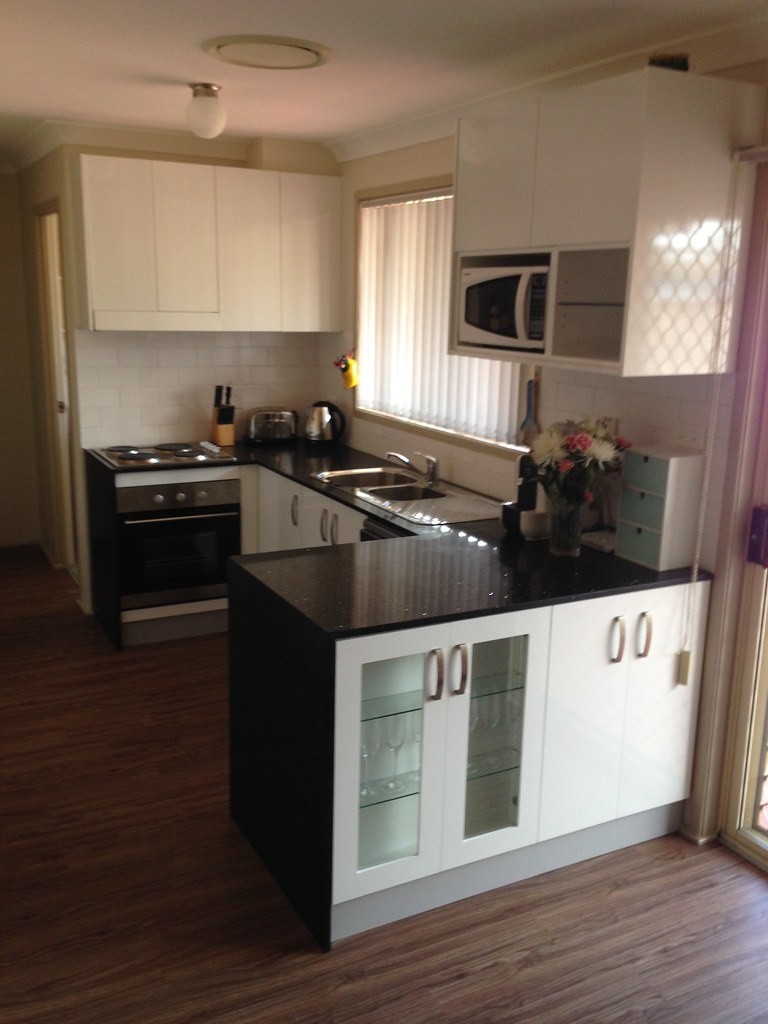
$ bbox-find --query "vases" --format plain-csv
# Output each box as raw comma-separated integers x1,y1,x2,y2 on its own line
546,497,585,558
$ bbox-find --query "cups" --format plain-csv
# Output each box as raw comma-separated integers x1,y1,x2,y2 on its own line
519,509,552,541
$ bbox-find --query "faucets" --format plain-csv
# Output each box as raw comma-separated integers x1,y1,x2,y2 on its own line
387,452,438,487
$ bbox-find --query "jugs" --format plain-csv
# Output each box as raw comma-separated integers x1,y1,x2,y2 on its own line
305,401,347,448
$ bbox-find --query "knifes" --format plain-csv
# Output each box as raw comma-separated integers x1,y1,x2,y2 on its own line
214,384,232,407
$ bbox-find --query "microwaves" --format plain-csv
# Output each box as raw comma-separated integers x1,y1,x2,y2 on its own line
456,265,550,351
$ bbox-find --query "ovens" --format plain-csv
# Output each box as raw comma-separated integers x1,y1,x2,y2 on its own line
107,479,244,622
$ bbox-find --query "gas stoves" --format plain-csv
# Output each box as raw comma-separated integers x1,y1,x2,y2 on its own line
92,441,237,470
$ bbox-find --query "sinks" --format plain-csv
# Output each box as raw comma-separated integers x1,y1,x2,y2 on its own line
360,483,445,501
312,465,421,490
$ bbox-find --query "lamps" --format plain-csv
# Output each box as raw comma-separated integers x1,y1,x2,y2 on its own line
185,84,225,138
201,34,334,72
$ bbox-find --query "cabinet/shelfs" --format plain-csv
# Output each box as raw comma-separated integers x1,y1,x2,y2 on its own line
537,579,711,844
256,467,367,553
61,154,345,335
333,606,554,906
452,63,765,375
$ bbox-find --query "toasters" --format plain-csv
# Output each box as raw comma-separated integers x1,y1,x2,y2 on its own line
242,406,299,451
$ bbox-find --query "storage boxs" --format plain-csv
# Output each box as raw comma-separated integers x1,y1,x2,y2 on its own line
614,446,703,570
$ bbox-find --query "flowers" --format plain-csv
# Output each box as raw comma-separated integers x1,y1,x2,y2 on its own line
532,419,633,544
